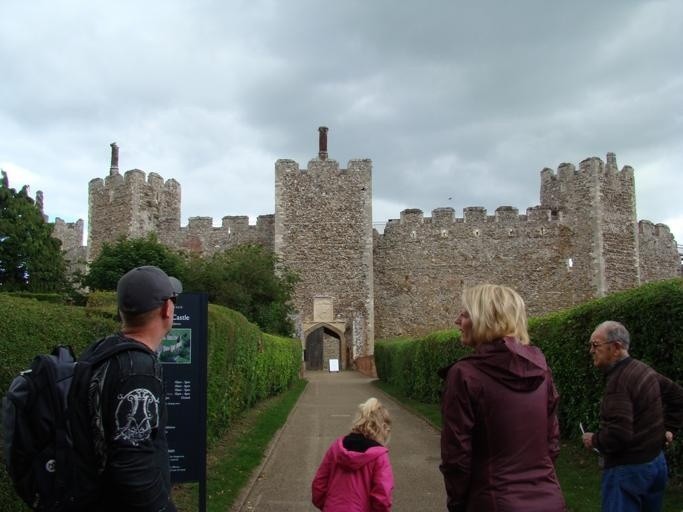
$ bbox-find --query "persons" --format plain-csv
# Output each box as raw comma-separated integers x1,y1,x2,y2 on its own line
68,264,183,511
440,284,565,510
578,320,682,510
309,396,395,511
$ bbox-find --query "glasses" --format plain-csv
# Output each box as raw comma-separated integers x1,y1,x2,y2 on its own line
589,339,616,349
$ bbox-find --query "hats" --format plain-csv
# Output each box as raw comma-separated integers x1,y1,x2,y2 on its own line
118,266,182,315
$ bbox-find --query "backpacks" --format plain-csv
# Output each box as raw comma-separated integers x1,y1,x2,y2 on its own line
3,337,157,511
